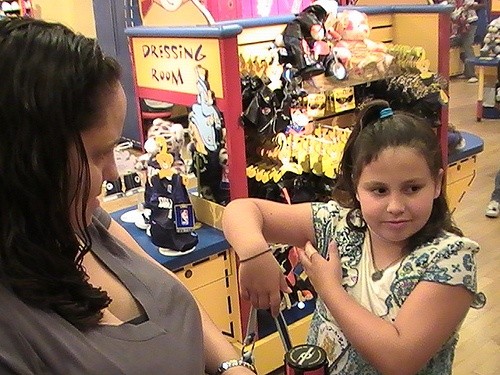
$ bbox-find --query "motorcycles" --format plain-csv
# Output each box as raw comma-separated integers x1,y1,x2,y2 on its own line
277,32,347,89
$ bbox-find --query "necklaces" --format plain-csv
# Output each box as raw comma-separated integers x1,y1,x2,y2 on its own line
367,228,409,282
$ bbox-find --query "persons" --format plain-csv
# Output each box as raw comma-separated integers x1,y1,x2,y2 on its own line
485,59,500,217
459,0,487,83
221,99,486,375
0,15,258,375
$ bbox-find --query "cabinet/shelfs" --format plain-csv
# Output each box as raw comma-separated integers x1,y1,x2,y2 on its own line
99,5,485,375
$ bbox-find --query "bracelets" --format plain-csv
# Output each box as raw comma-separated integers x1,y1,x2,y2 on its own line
217,358,257,374
239,248,272,262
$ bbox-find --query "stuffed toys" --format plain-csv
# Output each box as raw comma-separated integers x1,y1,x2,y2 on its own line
446,0,500,55
336,11,387,75
135,118,186,187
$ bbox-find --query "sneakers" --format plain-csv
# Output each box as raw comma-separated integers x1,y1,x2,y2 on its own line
484,200,500,216
